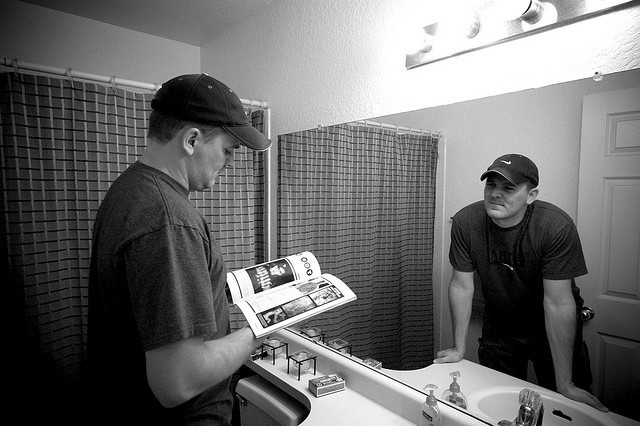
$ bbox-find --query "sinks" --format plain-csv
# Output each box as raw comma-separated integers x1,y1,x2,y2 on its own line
472,386,588,425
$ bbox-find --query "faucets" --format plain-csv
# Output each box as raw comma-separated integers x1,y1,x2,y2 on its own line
497,406,535,425
519,388,545,425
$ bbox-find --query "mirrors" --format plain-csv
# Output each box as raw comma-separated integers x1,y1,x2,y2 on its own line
278,66,640,426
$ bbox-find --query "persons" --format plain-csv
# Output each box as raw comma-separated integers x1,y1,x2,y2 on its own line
433,154,610,413
88,71,272,426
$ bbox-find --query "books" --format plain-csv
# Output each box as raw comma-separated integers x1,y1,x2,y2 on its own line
226,250,357,338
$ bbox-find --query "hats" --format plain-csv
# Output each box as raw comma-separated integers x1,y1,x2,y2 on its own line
151,73,273,152
480,154,539,185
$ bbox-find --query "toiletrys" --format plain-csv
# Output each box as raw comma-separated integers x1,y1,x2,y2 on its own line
443,369,467,408
415,384,440,425
